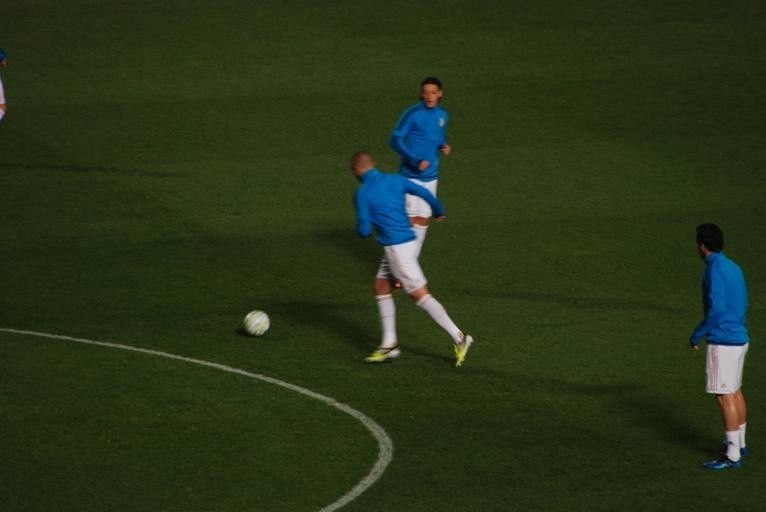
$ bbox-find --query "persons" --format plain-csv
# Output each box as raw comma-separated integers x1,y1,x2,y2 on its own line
351,151,474,367
389,77,451,289
689,223,750,470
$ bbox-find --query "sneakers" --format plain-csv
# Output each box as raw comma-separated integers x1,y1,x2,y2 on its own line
702,441,750,471
452,333,475,368
362,343,402,363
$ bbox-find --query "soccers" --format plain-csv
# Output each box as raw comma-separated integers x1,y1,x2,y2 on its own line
244,310,270,336
